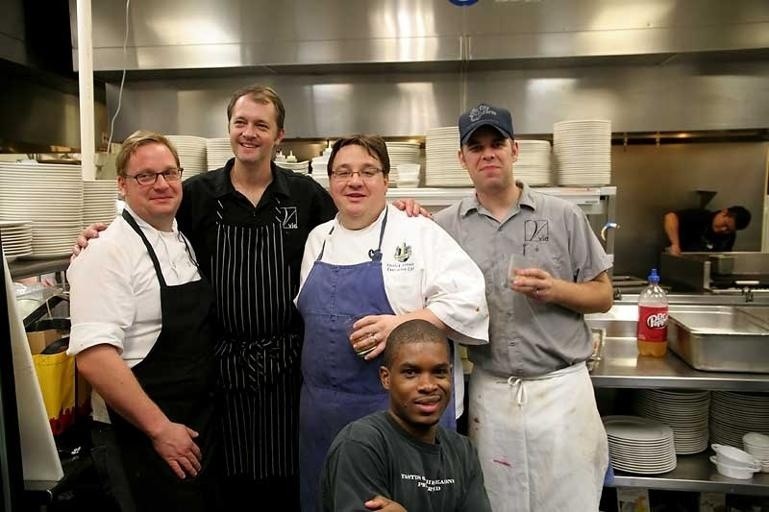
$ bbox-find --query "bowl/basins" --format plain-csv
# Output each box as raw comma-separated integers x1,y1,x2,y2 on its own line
709,444,761,480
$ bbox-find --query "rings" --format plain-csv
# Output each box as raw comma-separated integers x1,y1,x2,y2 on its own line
536,290,539,296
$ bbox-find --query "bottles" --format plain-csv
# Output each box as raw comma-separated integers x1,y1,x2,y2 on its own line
637,269,668,357
276,149,298,164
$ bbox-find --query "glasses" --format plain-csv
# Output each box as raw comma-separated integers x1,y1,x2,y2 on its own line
329,166,384,178
125,168,184,185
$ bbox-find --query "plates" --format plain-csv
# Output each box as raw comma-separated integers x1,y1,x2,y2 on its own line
742,432,769,473
163,135,207,183
384,141,420,187
311,156,332,189
82,180,118,230
639,390,710,454
274,161,309,175
425,127,476,188
512,140,552,187
600,415,677,475
0,161,84,261
553,120,611,187
205,138,235,171
711,390,769,451
1,221,33,264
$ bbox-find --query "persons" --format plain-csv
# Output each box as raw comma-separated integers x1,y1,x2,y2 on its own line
294,134,489,511
393,103,613,512
72,84,432,512
662,206,752,257
66,128,216,511
320,319,491,510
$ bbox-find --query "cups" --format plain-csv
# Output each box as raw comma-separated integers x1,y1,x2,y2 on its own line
342,311,379,358
507,254,541,289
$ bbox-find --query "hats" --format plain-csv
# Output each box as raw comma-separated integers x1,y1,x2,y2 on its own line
458,104,514,147
